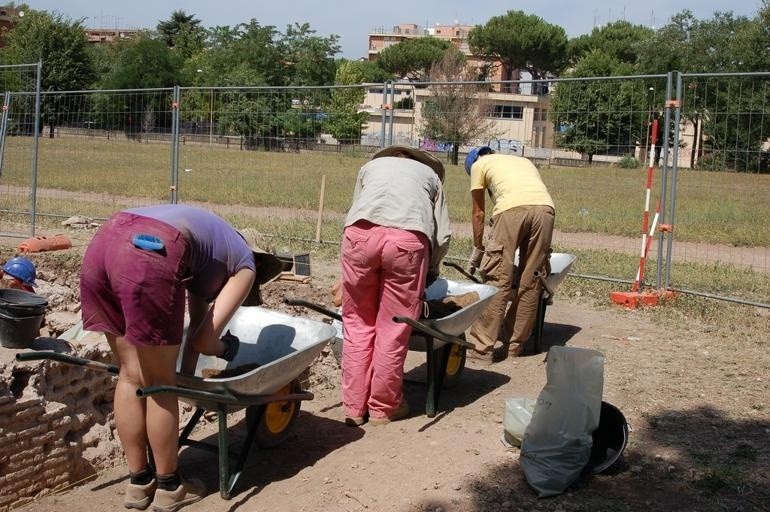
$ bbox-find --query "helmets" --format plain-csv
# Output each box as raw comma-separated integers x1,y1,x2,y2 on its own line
465,146,493,176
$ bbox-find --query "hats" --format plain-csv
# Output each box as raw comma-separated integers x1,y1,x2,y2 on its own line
235,226,285,284
372,144,445,185
3,256,38,288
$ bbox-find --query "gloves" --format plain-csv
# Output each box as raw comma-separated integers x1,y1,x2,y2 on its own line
466,247,485,275
217,329,240,362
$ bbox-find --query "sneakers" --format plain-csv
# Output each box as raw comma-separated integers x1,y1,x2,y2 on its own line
466,353,494,366
152,473,207,512
369,398,409,427
125,478,155,510
345,415,368,426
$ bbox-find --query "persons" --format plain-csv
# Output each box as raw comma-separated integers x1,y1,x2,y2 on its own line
465,145,555,361
340,143,451,425
1,259,37,292
80,203,281,512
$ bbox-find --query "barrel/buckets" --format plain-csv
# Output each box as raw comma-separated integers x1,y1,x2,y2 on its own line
584,400,629,474
0,289,48,349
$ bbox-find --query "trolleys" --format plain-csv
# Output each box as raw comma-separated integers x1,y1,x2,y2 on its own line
442,250,576,354
15,304,338,500
285,281,500,418
